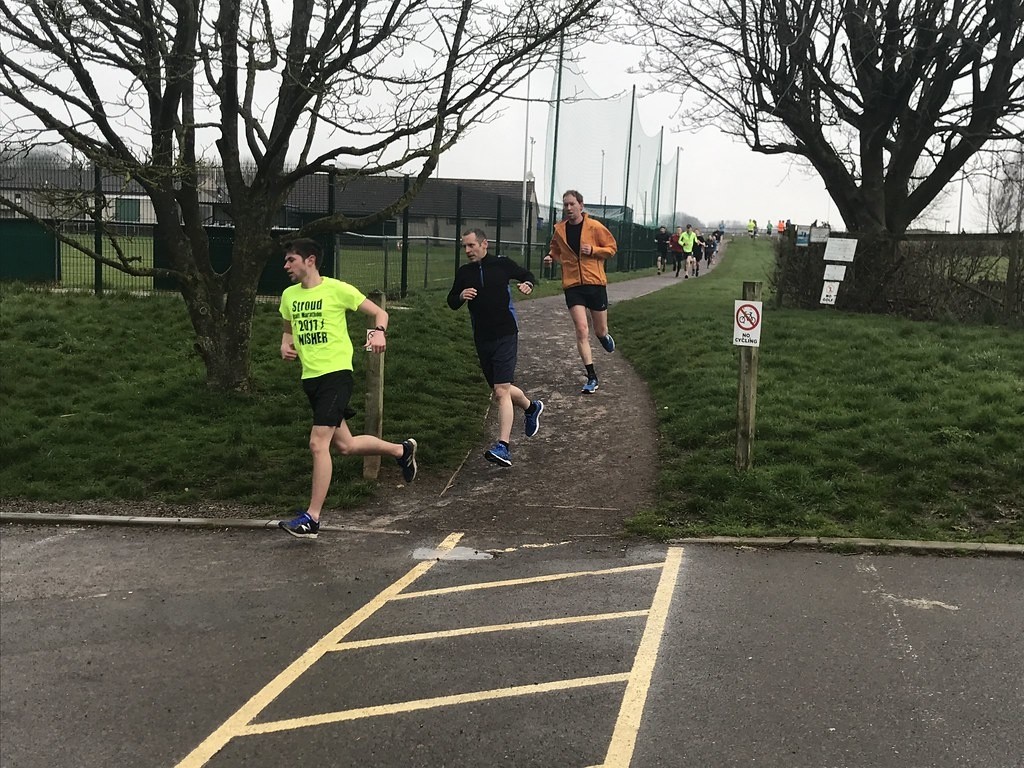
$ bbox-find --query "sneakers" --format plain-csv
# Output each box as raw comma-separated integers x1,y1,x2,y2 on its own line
582,377,599,394
523,401,544,437
598,334,615,353
484,443,513,467
396,438,418,483
279,512,320,539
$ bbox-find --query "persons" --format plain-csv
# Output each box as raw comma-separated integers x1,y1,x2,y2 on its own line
692,228,705,277
446,228,543,467
778,220,791,239
719,220,725,239
669,226,683,277
767,220,772,237
544,190,617,394
704,229,722,269
678,224,699,279
654,226,670,275
277,240,417,539
747,219,758,238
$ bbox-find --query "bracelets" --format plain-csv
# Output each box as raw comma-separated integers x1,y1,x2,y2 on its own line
545,254,552,258
590,246,593,255
525,283,532,289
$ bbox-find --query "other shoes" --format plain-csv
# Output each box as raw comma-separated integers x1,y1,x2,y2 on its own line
684,275,688,279
673,264,676,271
676,272,679,277
657,270,661,275
707,265,710,269
691,270,695,276
695,271,699,277
662,267,666,273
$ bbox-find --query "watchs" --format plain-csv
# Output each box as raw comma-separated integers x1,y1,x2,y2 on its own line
375,325,385,331
